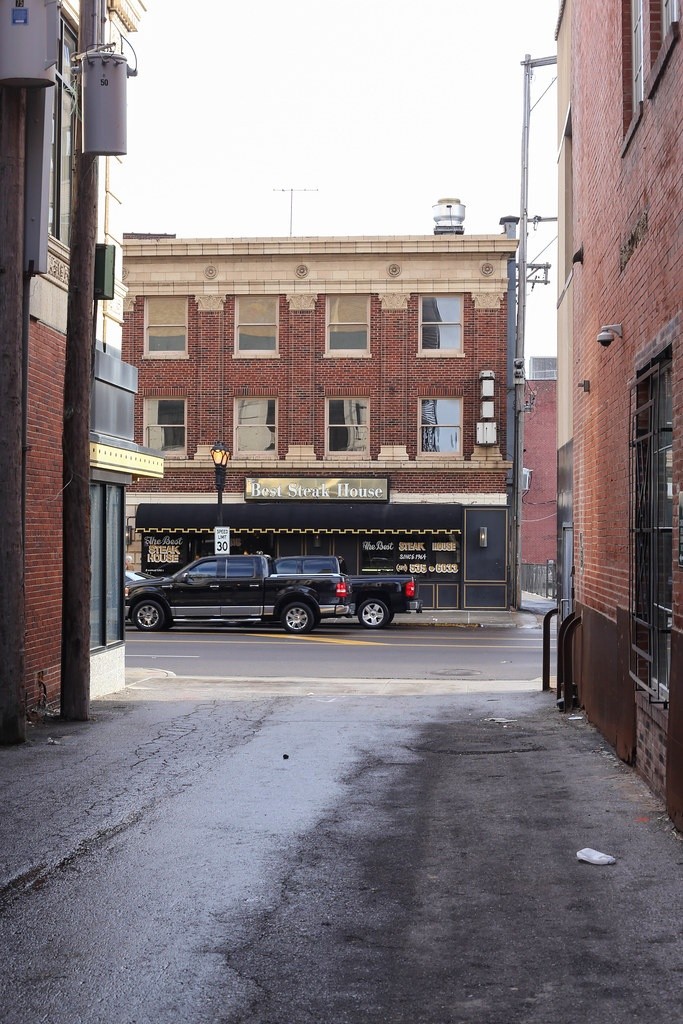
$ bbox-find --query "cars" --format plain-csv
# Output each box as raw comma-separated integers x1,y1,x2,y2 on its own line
123,570,158,582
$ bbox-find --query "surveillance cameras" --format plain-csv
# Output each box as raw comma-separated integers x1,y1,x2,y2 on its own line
597,331,614,346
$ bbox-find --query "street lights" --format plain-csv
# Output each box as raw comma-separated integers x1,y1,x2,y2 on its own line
210,440,230,526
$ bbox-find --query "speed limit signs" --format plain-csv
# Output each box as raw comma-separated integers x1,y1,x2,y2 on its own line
214,527,230,555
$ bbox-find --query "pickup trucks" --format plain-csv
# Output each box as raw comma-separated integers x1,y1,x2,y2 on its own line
270,554,423,631
124,553,355,634
